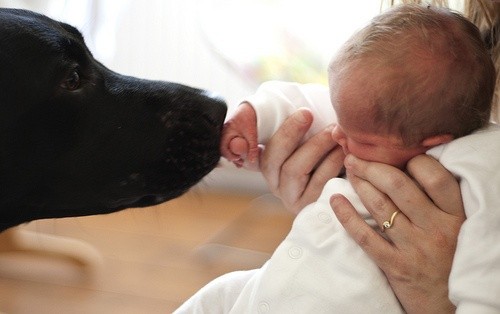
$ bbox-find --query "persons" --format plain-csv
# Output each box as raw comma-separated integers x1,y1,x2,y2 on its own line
172,8,500,314
219,1,500,314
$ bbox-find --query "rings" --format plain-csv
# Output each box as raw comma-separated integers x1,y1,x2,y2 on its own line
381,209,398,231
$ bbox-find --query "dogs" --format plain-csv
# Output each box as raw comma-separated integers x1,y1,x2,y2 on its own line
0,7,227,234
